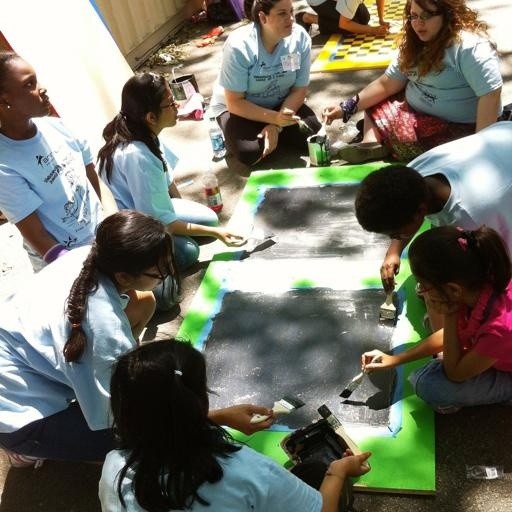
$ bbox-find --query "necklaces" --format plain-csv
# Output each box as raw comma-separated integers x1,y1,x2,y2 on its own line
307,0,391,35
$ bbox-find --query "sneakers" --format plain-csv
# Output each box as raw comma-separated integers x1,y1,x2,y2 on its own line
295,12,311,32
1,445,45,469
338,141,388,163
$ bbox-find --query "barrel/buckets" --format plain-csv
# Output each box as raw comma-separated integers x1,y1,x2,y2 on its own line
169,64,200,102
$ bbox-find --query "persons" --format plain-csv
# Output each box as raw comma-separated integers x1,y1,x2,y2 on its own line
95,71,246,270
355,119,512,296
320,0,505,164
222,0,319,33
96,337,373,512
0,208,183,471
0,51,182,313
361,226,511,418
209,0,323,169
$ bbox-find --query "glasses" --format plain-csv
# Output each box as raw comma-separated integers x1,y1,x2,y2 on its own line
143,260,174,279
415,282,439,295
161,96,175,109
408,10,443,20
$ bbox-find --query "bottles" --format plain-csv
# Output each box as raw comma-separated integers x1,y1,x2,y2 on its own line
203,169,223,213
208,116,227,158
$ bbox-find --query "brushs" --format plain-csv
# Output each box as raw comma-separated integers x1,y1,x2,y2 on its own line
380,277,396,319
232,228,274,240
249,394,305,425
284,107,314,135
311,109,329,142
317,403,370,469
339,356,382,398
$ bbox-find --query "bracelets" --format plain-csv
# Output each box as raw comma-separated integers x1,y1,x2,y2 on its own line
326,470,344,480
339,93,360,123
43,243,71,264
189,223,193,236
274,125,284,133
